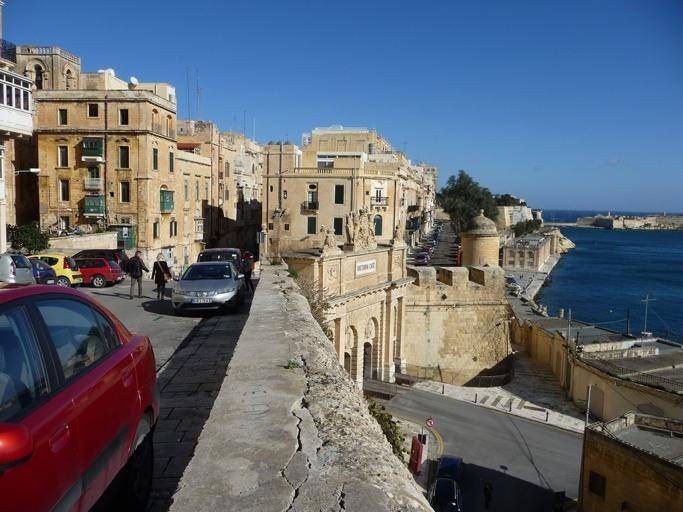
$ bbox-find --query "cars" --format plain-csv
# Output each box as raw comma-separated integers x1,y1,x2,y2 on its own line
169,260,245,316
427,478,463,512
414,218,443,266
435,454,464,488
503,272,520,290
0,280,160,511
70,258,122,288
26,258,56,286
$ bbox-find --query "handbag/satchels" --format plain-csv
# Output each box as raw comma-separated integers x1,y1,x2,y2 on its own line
164,273,172,281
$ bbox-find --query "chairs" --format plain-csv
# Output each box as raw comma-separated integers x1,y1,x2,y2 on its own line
191,269,202,280
51,331,102,373
0,344,26,421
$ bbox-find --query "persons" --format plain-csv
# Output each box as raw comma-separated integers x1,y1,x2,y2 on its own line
318,207,376,248
126,250,149,299
241,251,254,292
150,252,172,302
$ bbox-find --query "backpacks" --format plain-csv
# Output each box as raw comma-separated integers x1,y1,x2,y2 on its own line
129,257,140,273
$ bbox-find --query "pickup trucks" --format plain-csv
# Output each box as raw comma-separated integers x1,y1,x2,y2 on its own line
197,247,248,292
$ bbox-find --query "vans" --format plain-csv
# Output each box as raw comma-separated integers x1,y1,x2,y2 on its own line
24,252,82,289
69,248,130,284
0,253,36,286
